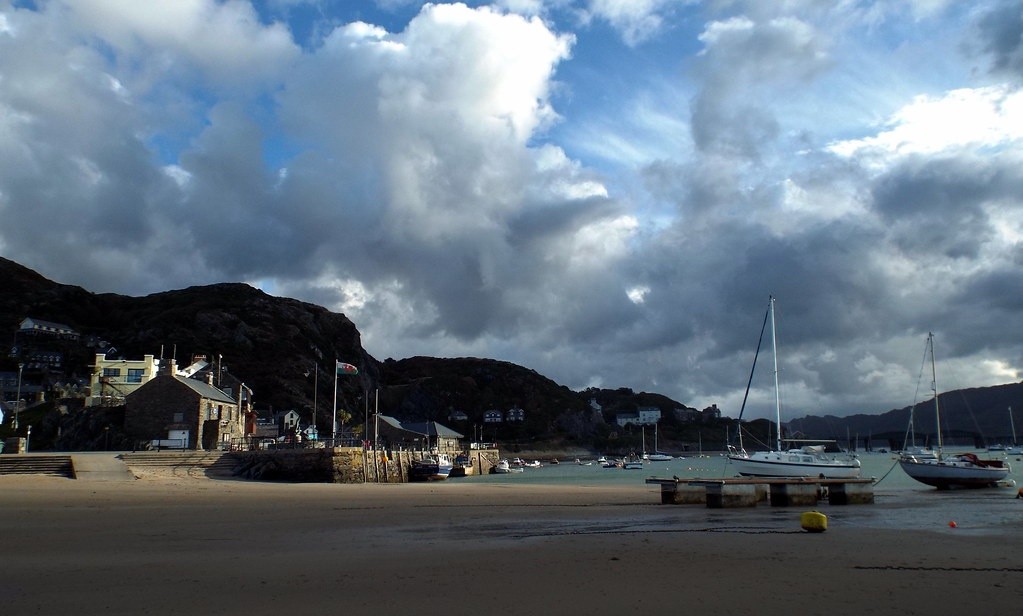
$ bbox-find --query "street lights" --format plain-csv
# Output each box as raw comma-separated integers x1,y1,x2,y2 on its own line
13,362,26,434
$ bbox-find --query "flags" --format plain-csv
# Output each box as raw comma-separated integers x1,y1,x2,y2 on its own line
337,362,358,375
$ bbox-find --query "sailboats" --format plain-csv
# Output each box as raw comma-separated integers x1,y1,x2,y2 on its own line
894,332,1013,488
726,294,861,479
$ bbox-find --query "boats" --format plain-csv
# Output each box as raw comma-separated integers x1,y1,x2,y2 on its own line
452,450,475,476
550,458,560,465
494,456,544,474
575,452,675,469
410,454,455,480
